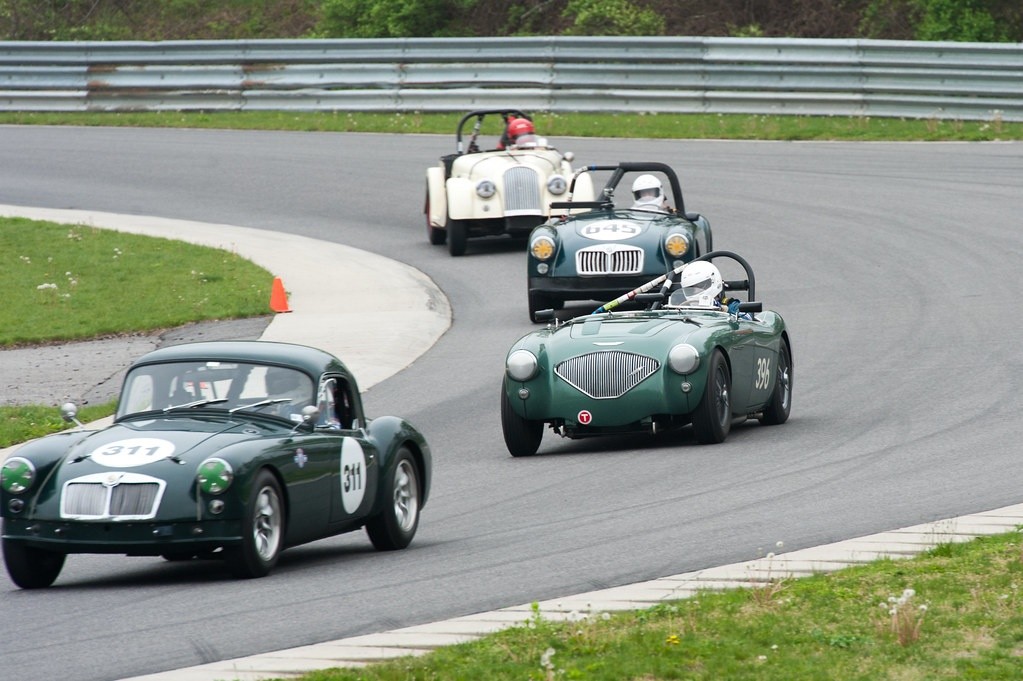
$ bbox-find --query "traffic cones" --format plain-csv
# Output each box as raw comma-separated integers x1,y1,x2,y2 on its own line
270,277,293,313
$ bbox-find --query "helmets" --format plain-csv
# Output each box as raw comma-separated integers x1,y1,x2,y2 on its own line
265,364,313,408
508,118,533,143
681,261,721,306
631,176,664,208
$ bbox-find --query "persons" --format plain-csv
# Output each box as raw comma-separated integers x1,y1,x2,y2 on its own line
680,260,753,320
632,175,671,212
266,370,341,431
509,118,536,150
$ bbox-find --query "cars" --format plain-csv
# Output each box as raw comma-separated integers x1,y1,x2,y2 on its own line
501,250,794,457
425,108,595,256
0,340,431,591
527,163,712,324
